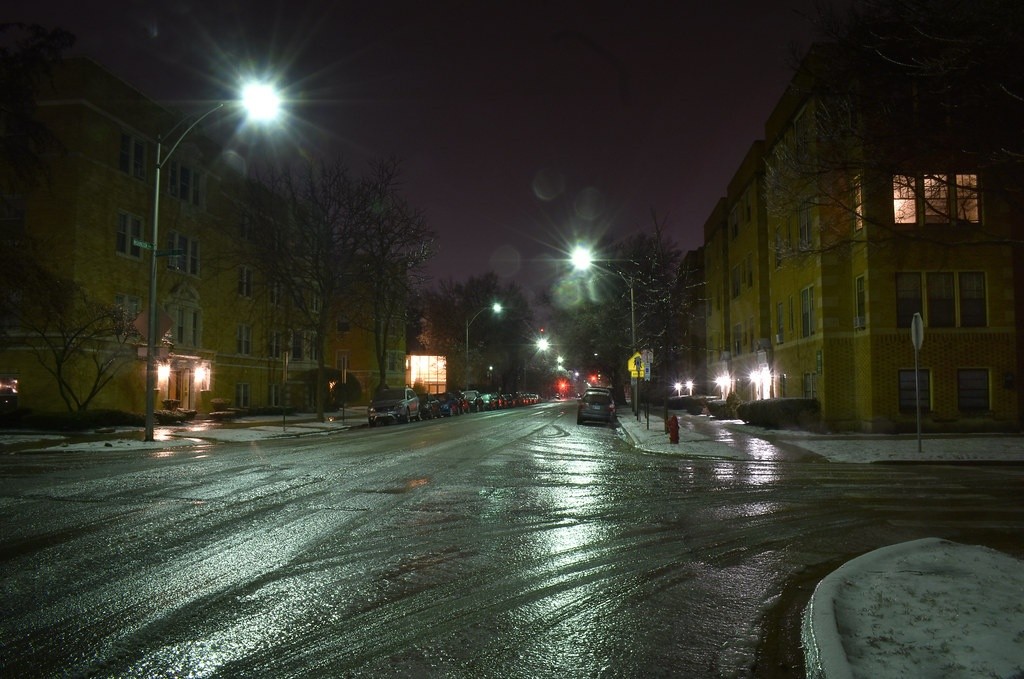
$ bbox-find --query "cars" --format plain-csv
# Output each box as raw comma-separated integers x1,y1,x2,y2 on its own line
480,392,544,411
416,393,441,419
456,391,470,414
576,391,615,425
465,390,485,412
433,393,460,416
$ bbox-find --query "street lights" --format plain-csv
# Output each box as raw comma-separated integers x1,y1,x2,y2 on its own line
570,247,640,420
465,303,503,390
143,82,284,442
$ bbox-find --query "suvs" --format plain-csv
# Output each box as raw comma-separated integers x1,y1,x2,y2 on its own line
366,386,421,427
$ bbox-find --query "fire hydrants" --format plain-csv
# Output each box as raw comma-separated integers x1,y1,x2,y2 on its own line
666,415,681,444
